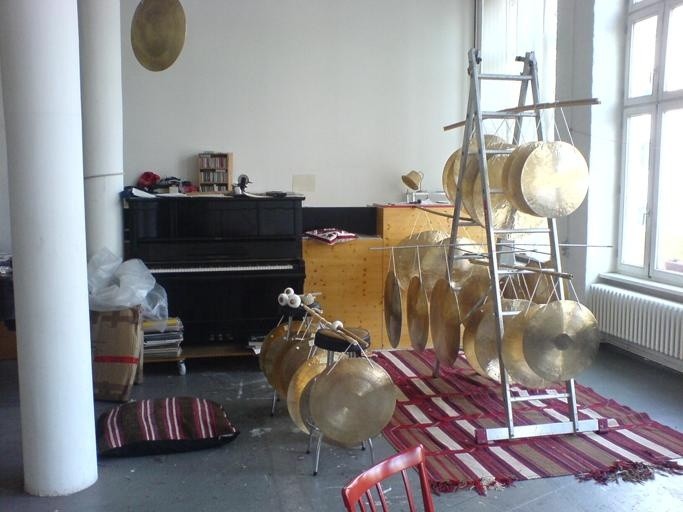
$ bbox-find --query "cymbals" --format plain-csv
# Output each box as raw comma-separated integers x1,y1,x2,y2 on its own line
130,0,187,70
441,134,589,234
260,320,397,445
382,230,599,389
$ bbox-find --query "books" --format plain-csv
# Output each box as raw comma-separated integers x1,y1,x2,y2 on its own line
197,151,233,195
141,316,185,360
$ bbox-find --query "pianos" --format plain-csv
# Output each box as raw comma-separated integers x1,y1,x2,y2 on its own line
120,187,306,348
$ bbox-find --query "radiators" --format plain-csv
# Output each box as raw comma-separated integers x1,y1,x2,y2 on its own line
589,283,683,359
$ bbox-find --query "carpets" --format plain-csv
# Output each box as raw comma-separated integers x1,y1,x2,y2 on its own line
346,345,682,495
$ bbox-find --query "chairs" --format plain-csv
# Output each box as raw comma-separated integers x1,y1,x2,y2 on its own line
340,442,435,512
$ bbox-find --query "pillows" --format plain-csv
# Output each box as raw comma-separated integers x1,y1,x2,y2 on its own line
89,304,240,457
